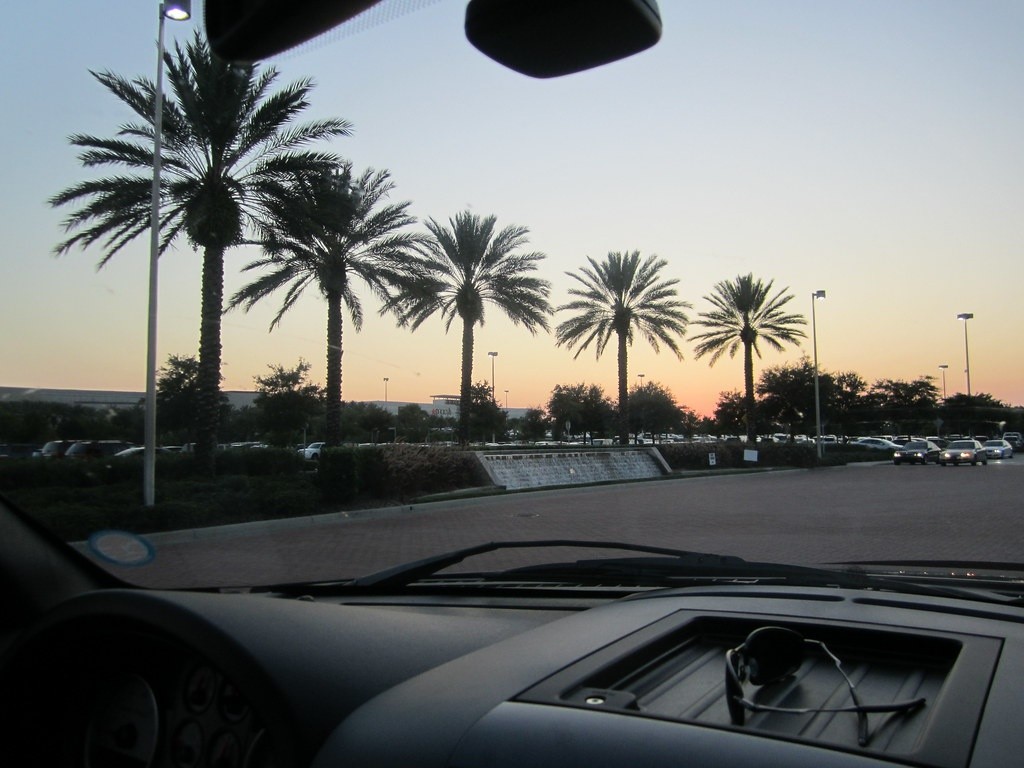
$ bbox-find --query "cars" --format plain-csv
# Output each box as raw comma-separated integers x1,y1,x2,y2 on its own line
34,433,1023,469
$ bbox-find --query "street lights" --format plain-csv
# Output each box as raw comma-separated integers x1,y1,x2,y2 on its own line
807,287,829,464
637,372,646,388
143,0,197,506
938,364,949,399
504,389,510,409
957,312,974,401
488,350,498,405
383,377,390,403
